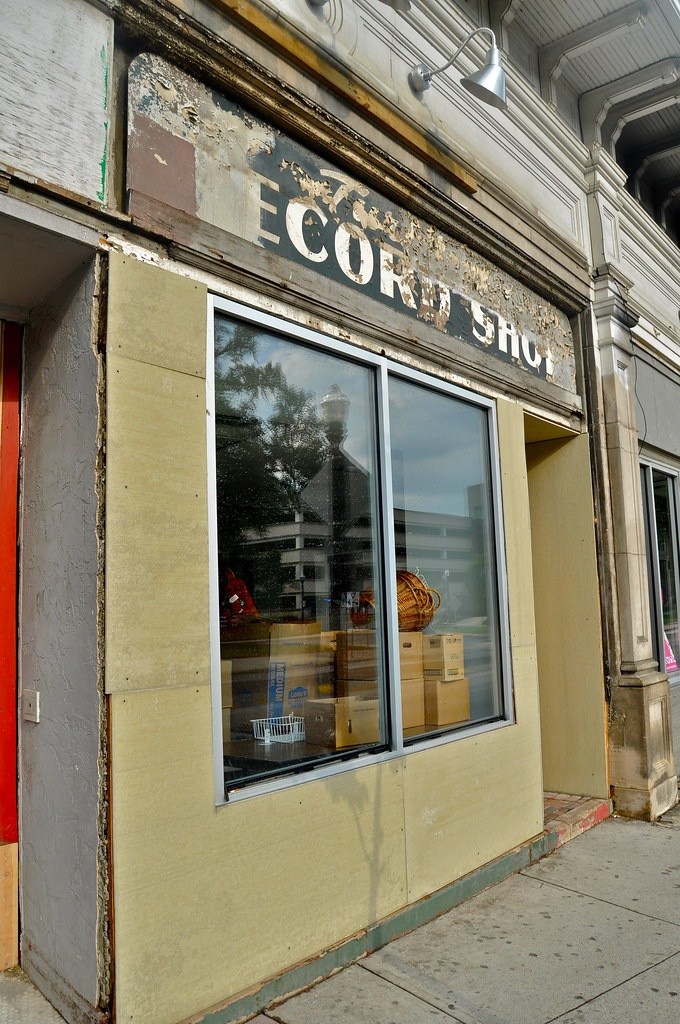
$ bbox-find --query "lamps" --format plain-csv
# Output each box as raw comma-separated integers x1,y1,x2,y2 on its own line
408,27,509,111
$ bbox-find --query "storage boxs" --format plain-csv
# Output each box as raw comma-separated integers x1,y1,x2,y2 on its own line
302,695,380,747
247,711,306,744
337,677,426,730
220,658,234,707
222,708,231,744
337,631,422,679
228,618,323,729
426,677,471,726
422,633,466,681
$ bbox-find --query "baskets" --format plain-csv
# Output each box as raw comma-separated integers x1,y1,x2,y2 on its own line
250,711,306,744
349,570,440,631
